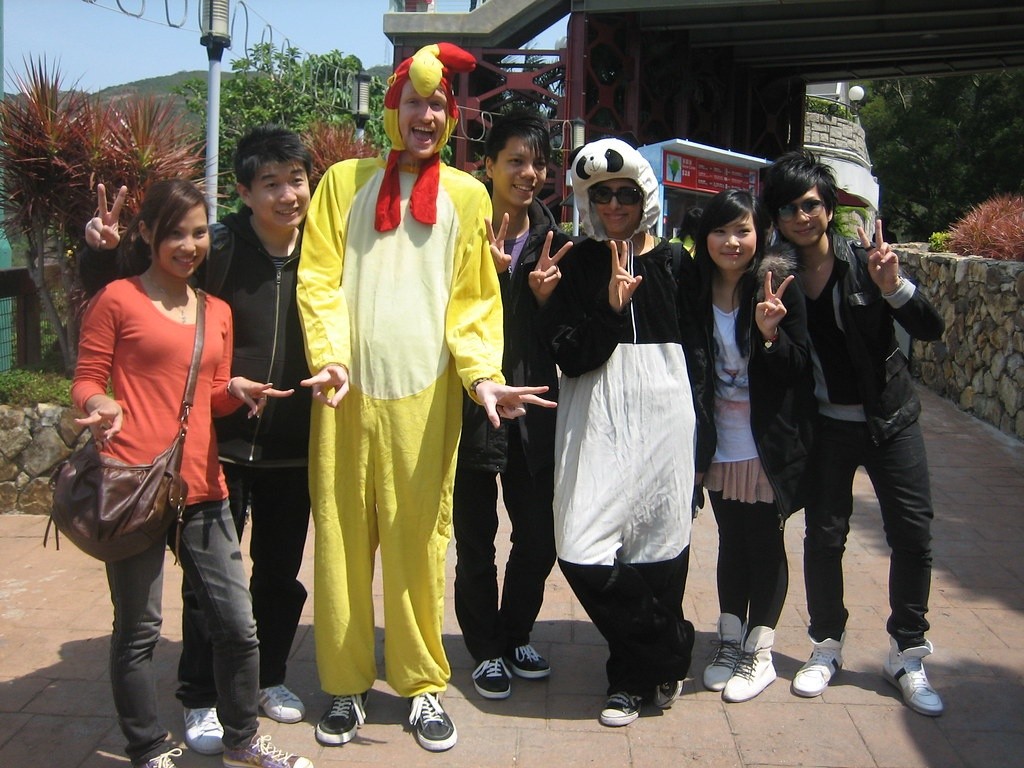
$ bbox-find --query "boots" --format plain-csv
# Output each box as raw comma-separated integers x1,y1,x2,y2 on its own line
724,624,776,702
702,613,747,691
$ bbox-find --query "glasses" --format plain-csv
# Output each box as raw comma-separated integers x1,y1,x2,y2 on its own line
778,200,825,222
586,185,642,205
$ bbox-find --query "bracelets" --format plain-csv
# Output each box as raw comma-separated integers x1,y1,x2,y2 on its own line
470,376,493,394
883,273,904,296
762,331,777,348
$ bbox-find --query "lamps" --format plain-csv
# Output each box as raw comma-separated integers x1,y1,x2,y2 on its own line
849,85,864,115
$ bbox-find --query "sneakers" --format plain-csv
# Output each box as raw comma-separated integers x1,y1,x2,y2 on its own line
257,685,306,723
653,678,683,707
472,655,512,699
502,643,552,678
601,691,642,726
315,690,368,744
881,635,943,716
221,732,313,768
183,706,225,754
408,691,457,751
792,625,846,697
131,748,182,768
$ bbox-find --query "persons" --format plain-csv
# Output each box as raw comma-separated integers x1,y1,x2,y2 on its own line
552,134,696,726
295,42,557,751
689,188,808,703
70,176,315,768
452,106,575,699
79,129,312,756
763,146,946,717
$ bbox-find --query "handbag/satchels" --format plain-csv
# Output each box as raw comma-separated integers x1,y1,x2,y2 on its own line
48,440,188,562
